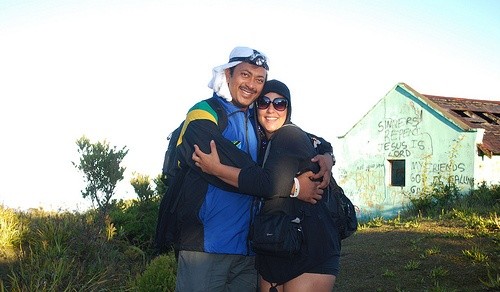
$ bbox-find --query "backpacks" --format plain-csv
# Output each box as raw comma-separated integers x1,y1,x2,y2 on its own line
161,98,228,188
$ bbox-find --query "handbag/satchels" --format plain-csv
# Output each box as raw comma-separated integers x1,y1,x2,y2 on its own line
337,187,358,240
248,208,305,258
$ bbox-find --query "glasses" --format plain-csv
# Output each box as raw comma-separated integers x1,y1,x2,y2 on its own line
256,96,289,111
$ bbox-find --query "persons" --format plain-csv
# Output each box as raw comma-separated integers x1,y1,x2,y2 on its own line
192,80,341,292
156,47,334,292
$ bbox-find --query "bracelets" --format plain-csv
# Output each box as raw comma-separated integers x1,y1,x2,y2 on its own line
329,153,335,165
290,177,300,197
